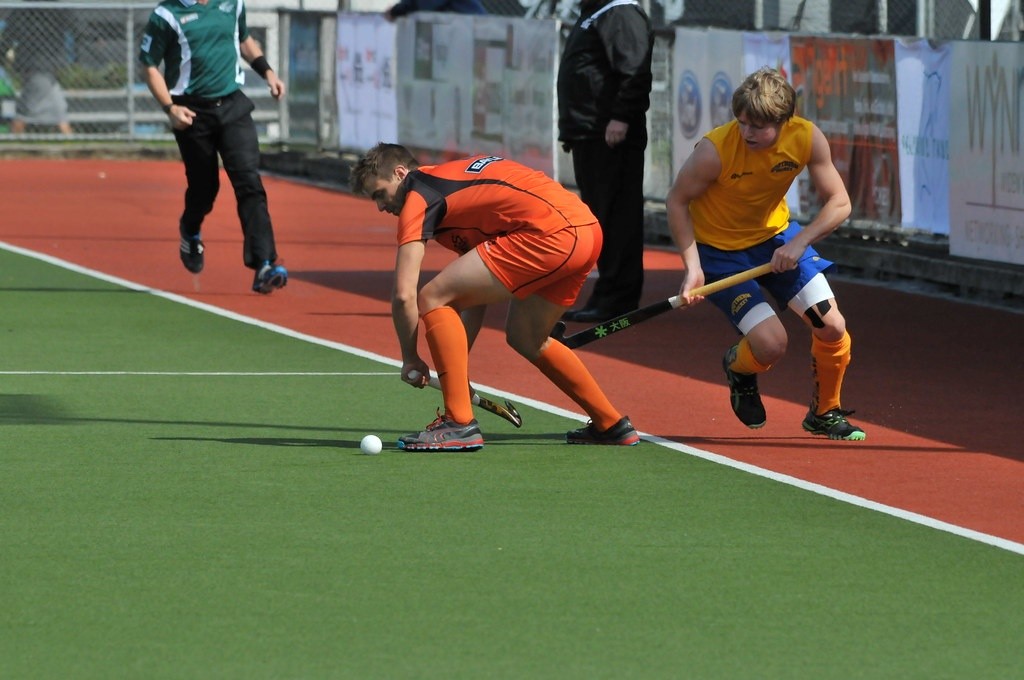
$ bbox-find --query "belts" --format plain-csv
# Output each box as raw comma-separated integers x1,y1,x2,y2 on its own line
189,97,227,108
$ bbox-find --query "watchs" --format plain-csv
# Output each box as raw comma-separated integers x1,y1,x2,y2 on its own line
162,104,173,113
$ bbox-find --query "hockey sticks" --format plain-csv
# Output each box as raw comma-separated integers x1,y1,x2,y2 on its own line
407,369,522,428
551,260,797,350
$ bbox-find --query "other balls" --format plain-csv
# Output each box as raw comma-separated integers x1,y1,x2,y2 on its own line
359,432,384,456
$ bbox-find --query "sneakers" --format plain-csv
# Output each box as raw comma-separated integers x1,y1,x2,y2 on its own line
398,407,484,452
252,260,288,295
179,218,204,274
723,344,767,429
566,415,640,446
561,305,623,322
802,400,866,442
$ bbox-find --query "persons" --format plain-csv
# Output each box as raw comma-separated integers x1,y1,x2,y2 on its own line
351,143,641,452
557,0,655,323
139,0,288,295
0,0,75,134
666,69,868,441
